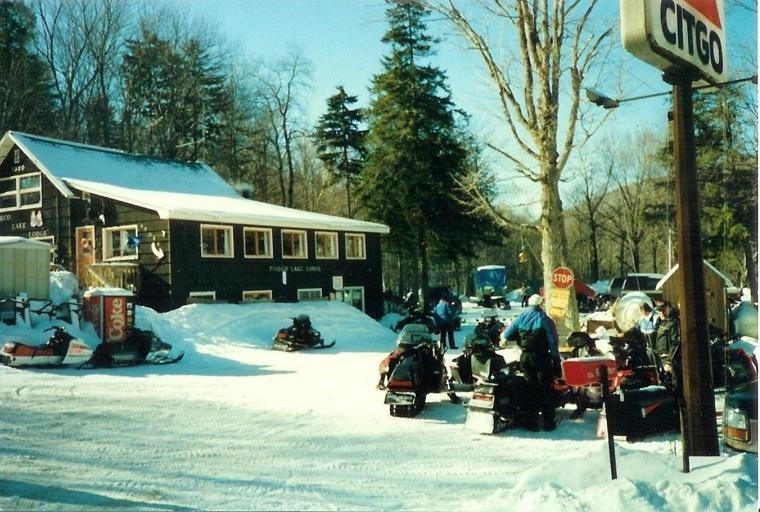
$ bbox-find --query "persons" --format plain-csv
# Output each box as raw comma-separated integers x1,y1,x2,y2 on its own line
521,283,529,307
639,301,683,386
504,294,562,432
433,297,458,349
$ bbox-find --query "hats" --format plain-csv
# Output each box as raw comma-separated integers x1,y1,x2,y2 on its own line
527,294,545,305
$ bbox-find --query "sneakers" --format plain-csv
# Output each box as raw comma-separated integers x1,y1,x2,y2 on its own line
450,346,458,348
525,423,556,431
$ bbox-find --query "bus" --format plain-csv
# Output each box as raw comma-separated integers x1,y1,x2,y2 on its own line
474,264,507,298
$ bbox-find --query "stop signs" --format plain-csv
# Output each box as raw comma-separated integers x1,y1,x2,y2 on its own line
552,266,576,290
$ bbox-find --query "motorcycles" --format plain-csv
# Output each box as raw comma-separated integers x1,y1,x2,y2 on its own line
272,315,335,352
0,326,185,368
477,293,511,310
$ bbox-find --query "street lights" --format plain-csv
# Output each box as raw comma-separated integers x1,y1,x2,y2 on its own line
583,73,758,109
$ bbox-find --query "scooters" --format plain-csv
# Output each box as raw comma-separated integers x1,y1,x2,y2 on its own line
596,297,758,446
378,288,451,418
451,315,530,435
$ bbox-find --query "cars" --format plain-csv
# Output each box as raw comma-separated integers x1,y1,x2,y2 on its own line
429,285,461,329
723,381,758,455
608,273,662,302
